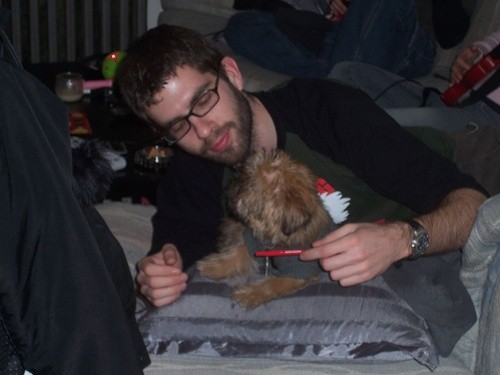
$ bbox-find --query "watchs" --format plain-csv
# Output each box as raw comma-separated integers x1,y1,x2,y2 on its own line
402,219,430,260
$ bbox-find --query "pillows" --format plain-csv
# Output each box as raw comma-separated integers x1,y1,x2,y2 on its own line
134,261,440,372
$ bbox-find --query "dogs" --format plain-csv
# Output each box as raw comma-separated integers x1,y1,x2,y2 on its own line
196,146,338,312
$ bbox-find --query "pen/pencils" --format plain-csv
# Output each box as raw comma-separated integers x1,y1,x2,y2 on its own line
255,247,307,257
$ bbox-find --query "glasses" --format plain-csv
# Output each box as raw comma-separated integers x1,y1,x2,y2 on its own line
161,72,220,144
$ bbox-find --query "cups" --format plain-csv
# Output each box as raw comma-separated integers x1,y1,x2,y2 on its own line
54,71,84,103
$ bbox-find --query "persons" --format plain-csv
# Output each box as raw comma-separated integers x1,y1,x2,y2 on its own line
112,25,489,307
